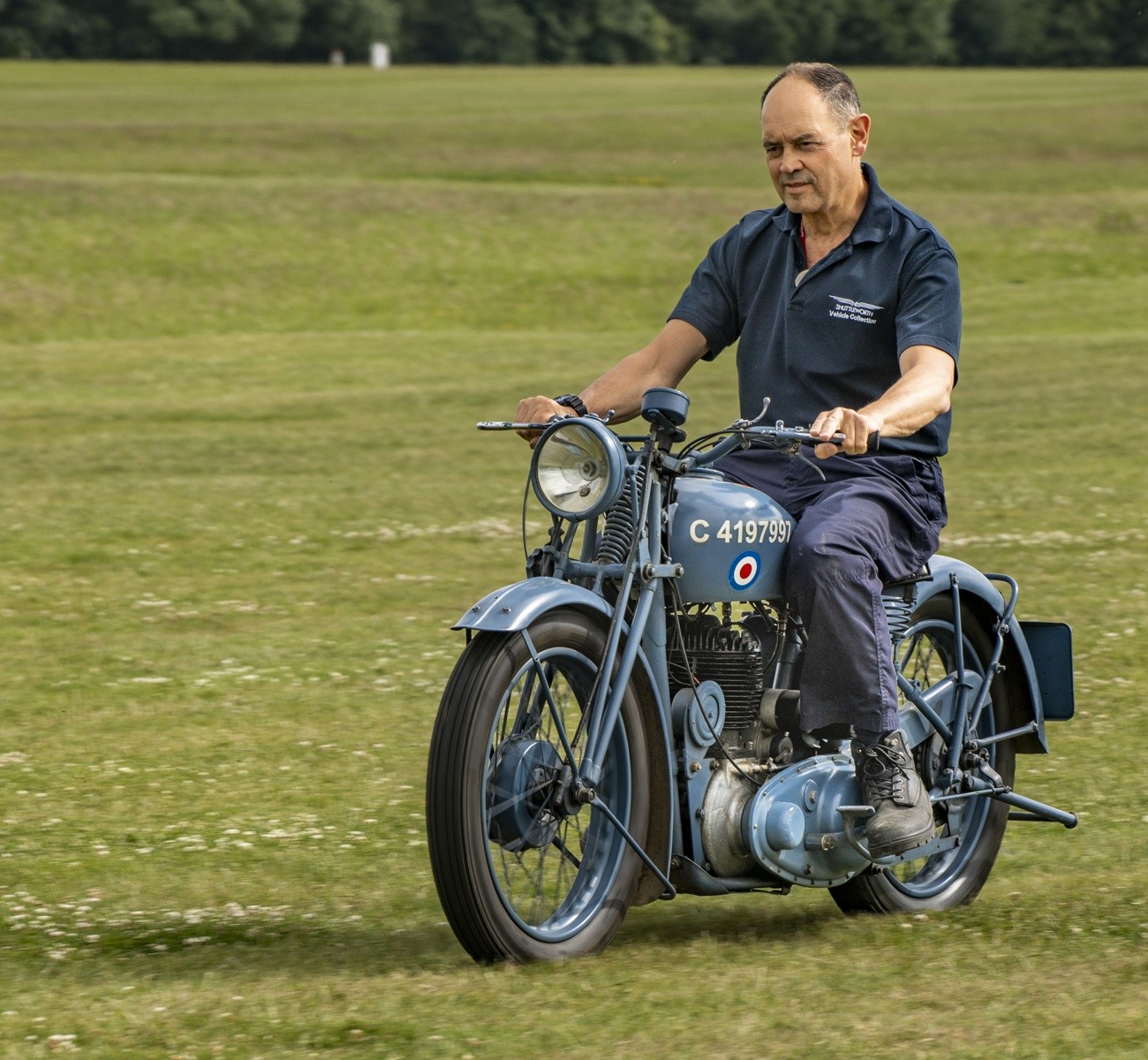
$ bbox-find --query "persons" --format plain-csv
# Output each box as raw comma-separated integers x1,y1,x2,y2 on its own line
513,62,961,854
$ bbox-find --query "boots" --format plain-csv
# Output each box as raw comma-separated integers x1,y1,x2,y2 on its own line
850,729,935,859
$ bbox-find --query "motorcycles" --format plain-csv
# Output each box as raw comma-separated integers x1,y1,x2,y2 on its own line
424,389,1080,971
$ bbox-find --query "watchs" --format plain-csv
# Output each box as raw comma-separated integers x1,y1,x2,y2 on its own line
553,393,588,417
